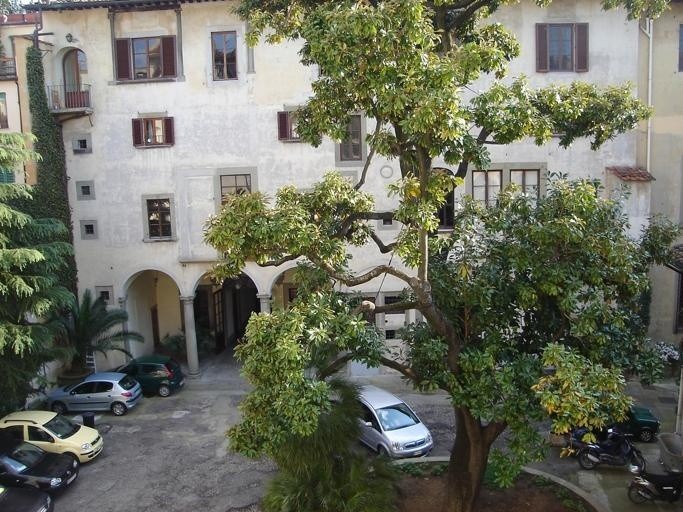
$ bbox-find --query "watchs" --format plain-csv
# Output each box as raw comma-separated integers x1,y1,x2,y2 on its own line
567,426,647,475
625,459,683,505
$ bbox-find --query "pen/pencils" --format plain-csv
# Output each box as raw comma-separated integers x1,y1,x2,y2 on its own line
339,384,434,460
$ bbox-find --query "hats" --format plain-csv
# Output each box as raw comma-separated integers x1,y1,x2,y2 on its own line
0,357,187,512
565,399,660,442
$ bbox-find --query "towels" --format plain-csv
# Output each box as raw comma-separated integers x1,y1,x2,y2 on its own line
82,412,94,428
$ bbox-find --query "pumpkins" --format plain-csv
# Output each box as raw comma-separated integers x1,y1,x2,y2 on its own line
85,351,96,373
